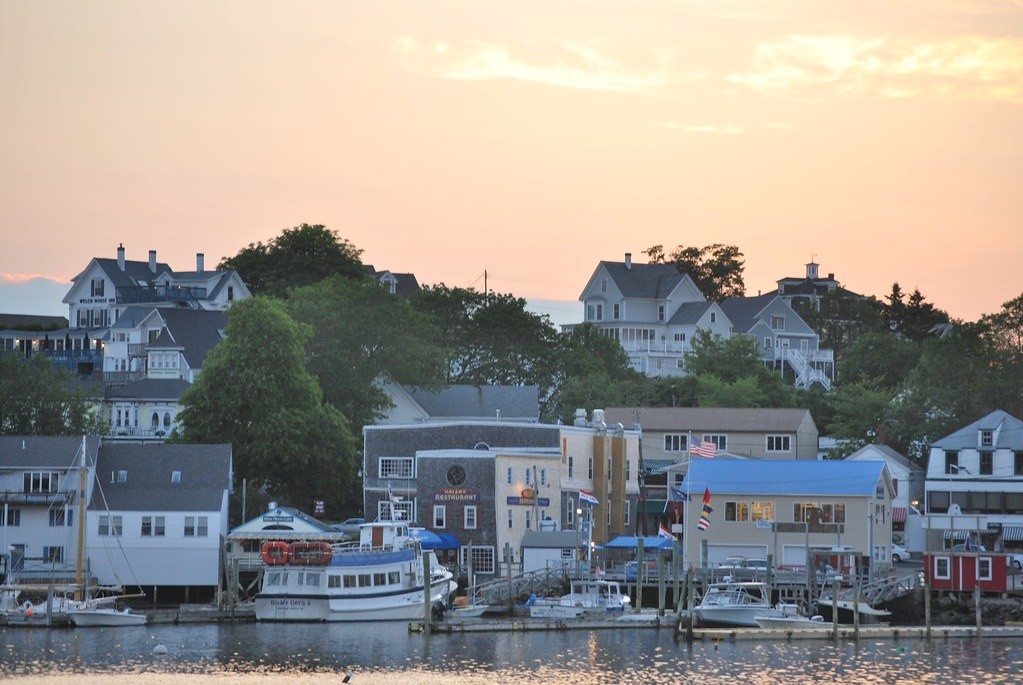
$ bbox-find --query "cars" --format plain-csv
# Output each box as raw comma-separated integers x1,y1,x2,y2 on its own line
746,558,775,577
954,544,985,551
717,557,746,569
328,518,365,532
891,543,911,563
1006,553,1023,569
634,553,658,570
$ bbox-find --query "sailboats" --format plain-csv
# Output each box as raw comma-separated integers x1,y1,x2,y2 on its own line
0,435,147,625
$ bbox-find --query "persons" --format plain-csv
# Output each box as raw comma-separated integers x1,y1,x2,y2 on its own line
22,606,30,623
526,551,636,624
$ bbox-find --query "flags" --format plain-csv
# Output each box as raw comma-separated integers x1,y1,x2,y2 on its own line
688,433,718,460
669,486,696,503
658,525,683,553
696,488,715,534
577,489,602,508
962,531,973,552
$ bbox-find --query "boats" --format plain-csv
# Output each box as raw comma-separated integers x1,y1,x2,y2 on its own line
254,481,460,622
752,604,834,628
526,578,632,619
695,575,798,626
443,575,489,617
67,602,148,626
817,600,893,625
